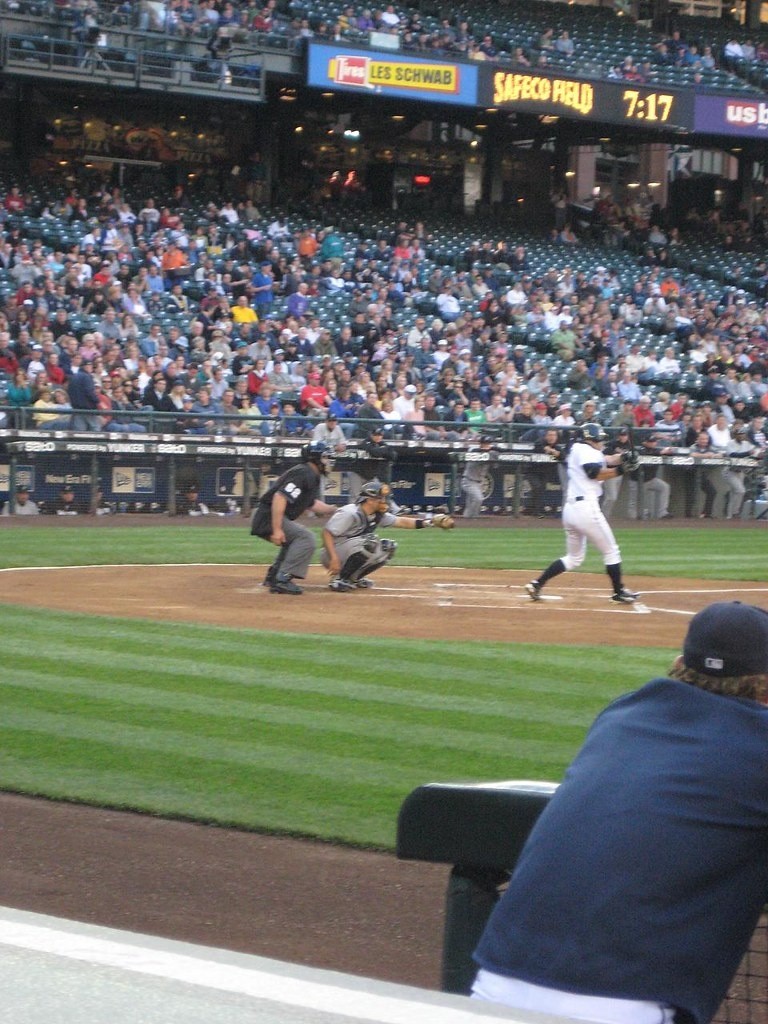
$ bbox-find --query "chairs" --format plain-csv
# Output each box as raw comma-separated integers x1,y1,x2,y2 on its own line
0,147,768,450
0,0,768,102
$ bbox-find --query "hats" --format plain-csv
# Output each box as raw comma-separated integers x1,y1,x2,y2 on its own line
185,484,199,493
647,435,657,442
327,413,337,420
681,601,767,677
60,485,74,494
437,339,448,345
270,401,279,409
618,428,629,435
404,384,418,393
14,484,30,493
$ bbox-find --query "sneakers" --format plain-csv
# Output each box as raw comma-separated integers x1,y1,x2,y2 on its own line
611,589,640,604
524,580,540,601
349,577,373,588
262,573,275,587
270,573,305,595
328,579,357,591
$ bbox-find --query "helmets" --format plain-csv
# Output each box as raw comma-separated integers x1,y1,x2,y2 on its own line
301,441,335,474
354,482,387,504
578,424,609,440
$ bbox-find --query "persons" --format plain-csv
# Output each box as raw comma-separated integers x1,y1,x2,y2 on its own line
320,479,455,592
0,0,768,516
249,441,341,593
524,422,641,604
464,599,768,1024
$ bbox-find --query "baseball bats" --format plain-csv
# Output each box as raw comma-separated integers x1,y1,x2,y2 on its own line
625,420,635,460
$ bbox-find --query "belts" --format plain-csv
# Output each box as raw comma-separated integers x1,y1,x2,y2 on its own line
576,496,584,501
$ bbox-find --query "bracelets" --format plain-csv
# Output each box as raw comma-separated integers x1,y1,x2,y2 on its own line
415,519,425,529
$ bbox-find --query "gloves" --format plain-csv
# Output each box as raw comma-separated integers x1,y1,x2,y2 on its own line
620,450,639,463
617,458,639,474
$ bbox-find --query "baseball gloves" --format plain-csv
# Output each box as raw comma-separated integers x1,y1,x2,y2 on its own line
425,513,456,530
